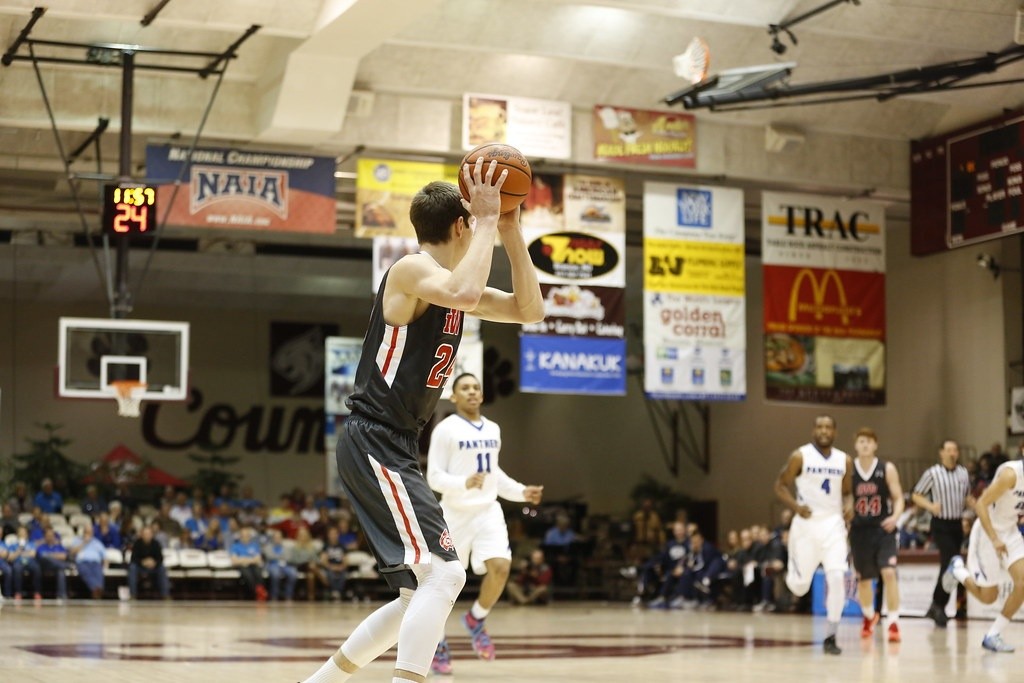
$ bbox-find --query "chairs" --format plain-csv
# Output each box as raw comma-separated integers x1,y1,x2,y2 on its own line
0,503,378,601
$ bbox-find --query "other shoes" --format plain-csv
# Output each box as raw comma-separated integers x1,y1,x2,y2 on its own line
630,595,779,613
929,603,947,623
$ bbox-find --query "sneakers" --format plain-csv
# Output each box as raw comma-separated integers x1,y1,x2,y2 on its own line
432,639,452,674
463,611,494,660
823,640,842,655
943,556,964,594
982,634,1015,653
861,613,879,638
888,623,900,642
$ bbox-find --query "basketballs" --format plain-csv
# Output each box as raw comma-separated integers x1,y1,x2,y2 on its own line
457,142,532,214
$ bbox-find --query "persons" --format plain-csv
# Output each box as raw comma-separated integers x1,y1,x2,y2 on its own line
851,427,904,642
0,478,367,600
427,372,544,675
299,155,545,683
911,440,977,627
961,443,1010,548
942,442,1024,653
632,511,792,612
778,414,854,655
508,550,552,605
544,515,574,544
